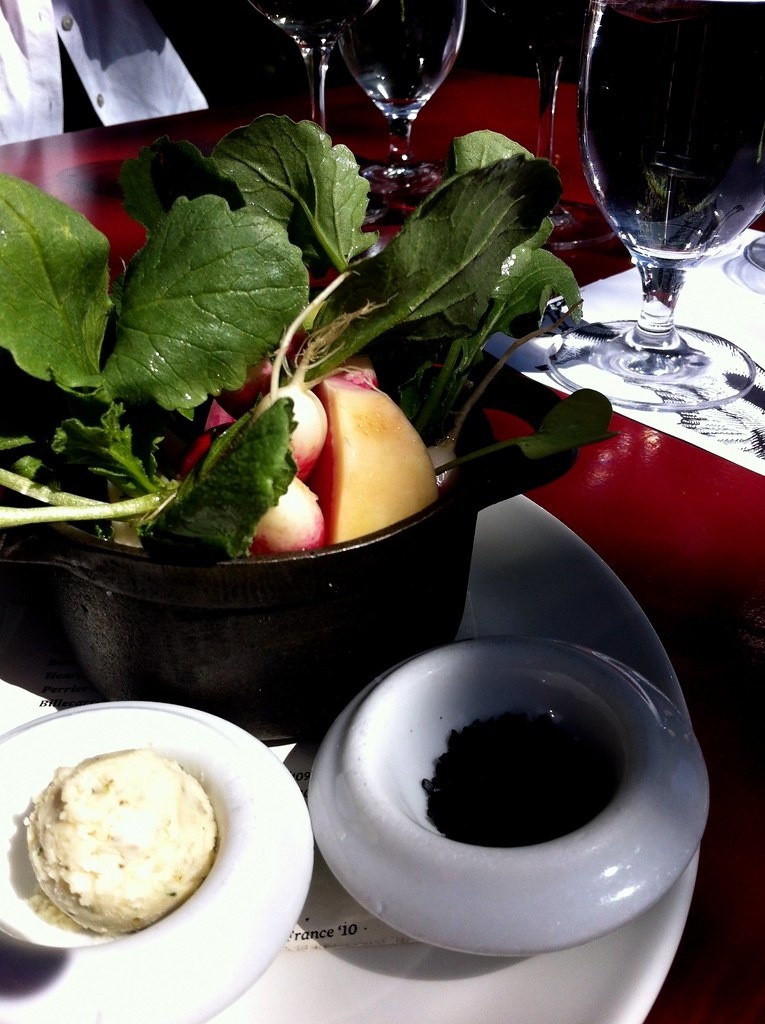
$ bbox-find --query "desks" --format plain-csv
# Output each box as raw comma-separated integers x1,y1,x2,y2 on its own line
0,73,765,1024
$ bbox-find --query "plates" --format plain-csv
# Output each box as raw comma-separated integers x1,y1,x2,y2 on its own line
311,633,710,959
0,701,315,1024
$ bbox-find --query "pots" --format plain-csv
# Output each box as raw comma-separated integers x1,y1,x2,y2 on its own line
0,352,579,735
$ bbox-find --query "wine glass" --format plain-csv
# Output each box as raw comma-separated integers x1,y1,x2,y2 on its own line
338,0,466,193
248,0,389,227
482,0,614,251
548,0,765,413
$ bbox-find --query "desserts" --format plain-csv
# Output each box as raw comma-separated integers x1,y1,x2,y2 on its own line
23,748,217,935
